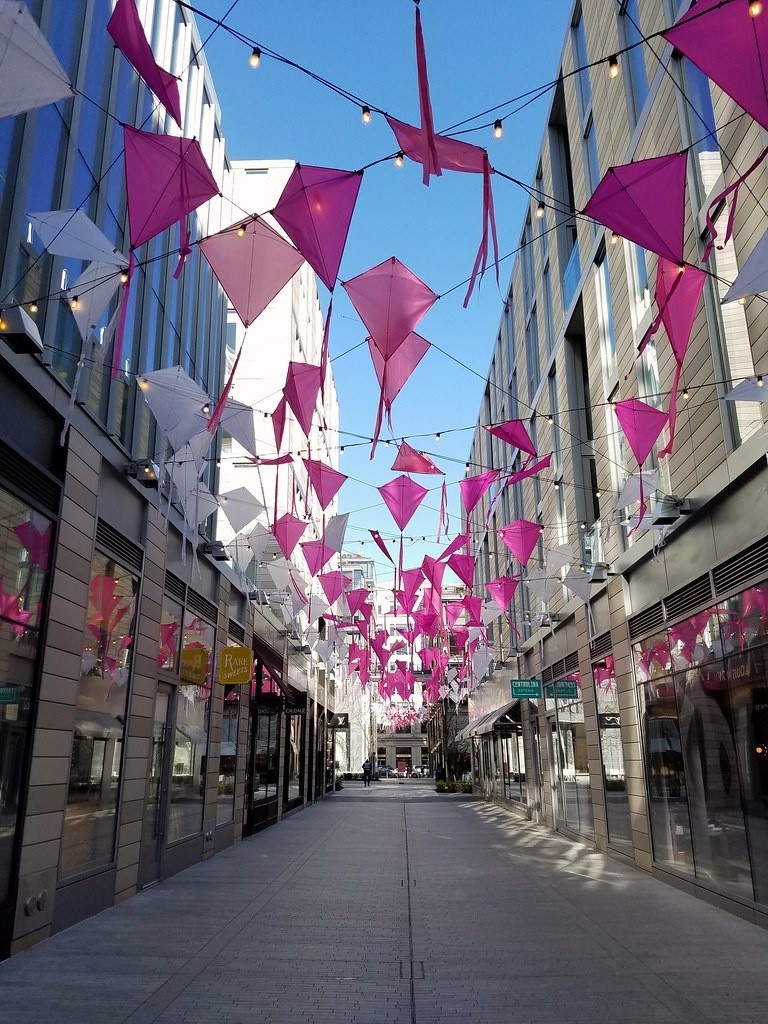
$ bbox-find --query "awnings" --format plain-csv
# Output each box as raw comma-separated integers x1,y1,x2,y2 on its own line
455,698,521,742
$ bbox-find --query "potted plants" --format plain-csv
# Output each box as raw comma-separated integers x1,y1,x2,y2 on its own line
434,780,472,794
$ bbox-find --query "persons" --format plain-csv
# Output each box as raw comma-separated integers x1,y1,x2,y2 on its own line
362,759,372,787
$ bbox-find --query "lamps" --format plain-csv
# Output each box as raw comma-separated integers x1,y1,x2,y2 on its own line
539,613,559,628
298,645,312,654
249,590,269,605
283,629,301,640
198,540,230,562
651,495,691,525
494,661,507,670
507,646,523,657
0,303,45,355
588,562,616,584
480,675,491,683
124,458,167,490
